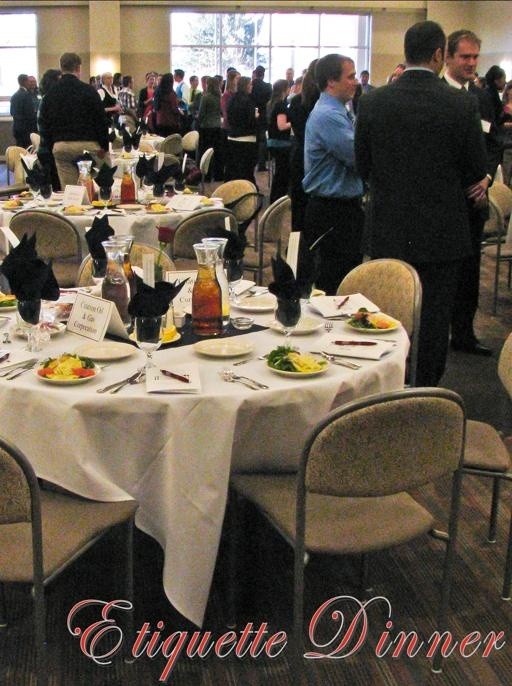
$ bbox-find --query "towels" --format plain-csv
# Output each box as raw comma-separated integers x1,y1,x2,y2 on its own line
2,229,60,324
123,270,189,341
266,253,303,326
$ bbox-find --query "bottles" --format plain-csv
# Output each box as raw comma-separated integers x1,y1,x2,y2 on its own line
191,238,230,335
101,234,135,325
121,160,139,204
77,160,94,203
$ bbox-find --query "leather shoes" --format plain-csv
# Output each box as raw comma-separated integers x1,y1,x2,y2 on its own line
449,336,494,357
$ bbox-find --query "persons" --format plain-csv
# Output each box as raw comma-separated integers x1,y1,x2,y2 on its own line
9,52,321,202
352,63,511,191
354,20,487,389
297,53,365,295
441,29,505,358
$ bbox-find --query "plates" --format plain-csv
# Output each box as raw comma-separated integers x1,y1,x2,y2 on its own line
345,314,400,332
231,294,276,312
194,338,255,359
270,317,323,335
15,321,65,338
0,192,215,217
36,342,137,385
265,356,330,378
0,305,18,313
310,291,380,317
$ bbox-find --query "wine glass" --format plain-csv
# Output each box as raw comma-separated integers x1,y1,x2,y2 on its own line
273,299,301,350
91,259,106,286
222,258,244,306
15,299,42,353
134,316,162,384
118,130,139,159
28,168,186,214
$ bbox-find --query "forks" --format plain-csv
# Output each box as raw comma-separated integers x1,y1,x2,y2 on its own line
325,320,333,333
311,350,361,369
0,358,37,379
221,367,268,391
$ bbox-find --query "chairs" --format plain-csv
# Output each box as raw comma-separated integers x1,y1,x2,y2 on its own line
462,334,512,601
336,258,422,388
0,105,295,286
477,180,511,317
1,436,138,685
221,386,465,674
